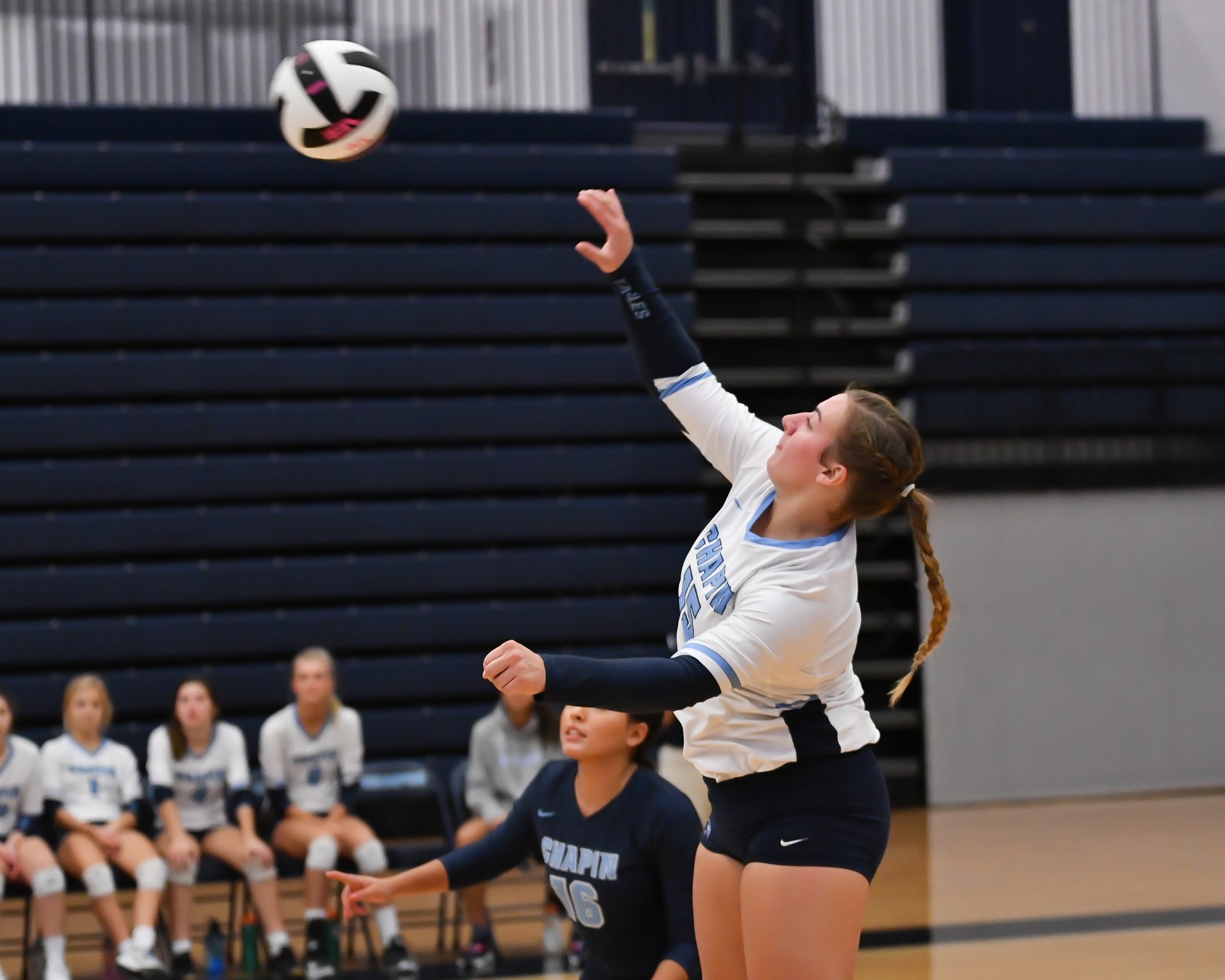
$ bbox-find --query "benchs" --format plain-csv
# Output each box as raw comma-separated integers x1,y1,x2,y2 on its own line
0,102,706,753
833,113,1224,447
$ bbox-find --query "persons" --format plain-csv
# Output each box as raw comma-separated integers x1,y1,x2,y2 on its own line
40,674,170,980
146,679,305,980
482,191,953,980
456,692,593,968
256,648,420,980
0,694,70,980
324,705,703,979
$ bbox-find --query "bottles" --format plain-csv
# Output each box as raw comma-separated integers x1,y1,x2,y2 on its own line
239,914,259,977
327,909,341,970
540,905,564,975
204,920,225,980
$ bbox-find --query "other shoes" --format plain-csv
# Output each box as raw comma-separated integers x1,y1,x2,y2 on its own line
267,942,295,970
381,936,418,980
115,949,168,976
565,928,583,968
172,945,209,979
305,922,337,980
455,936,498,969
43,959,69,980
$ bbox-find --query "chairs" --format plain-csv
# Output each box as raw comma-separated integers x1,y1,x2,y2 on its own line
0,761,476,979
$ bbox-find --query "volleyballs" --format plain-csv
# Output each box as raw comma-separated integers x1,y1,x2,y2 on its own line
267,39,400,160
